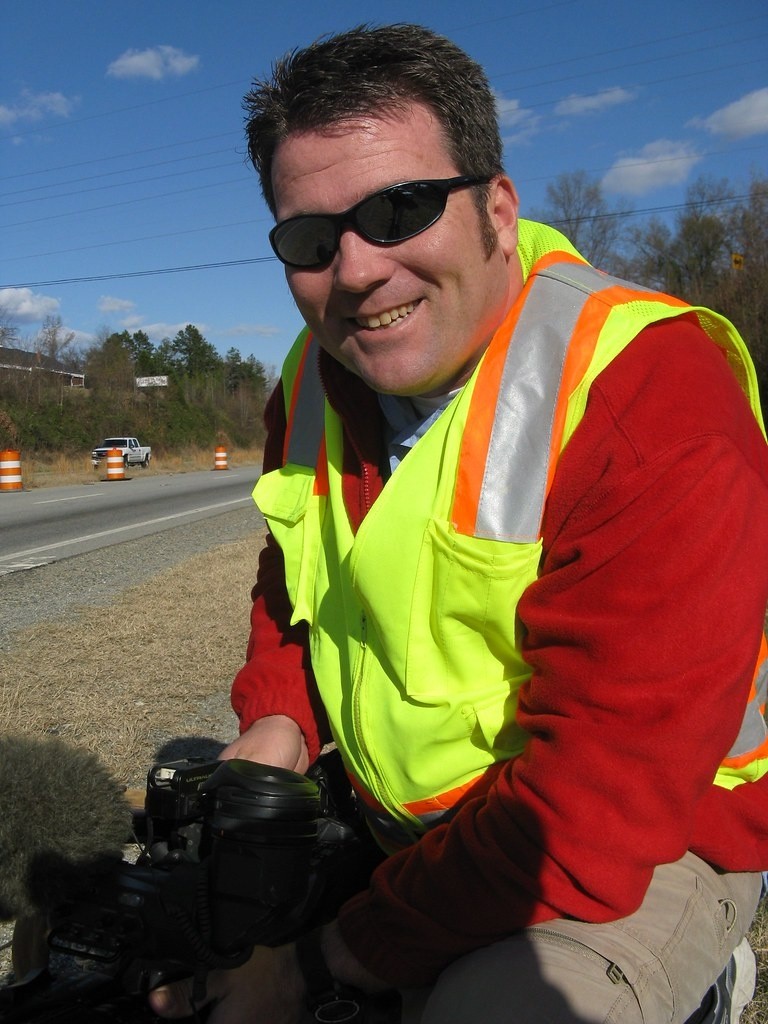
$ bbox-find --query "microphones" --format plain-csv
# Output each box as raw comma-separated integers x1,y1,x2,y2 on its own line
27,849,157,916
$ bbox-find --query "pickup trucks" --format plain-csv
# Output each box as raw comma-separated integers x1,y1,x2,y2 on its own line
92,437,152,470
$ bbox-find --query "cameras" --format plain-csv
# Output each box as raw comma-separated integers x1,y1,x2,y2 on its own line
134,754,365,1024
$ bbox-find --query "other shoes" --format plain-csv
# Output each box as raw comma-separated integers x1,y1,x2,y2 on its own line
700,935,757,1024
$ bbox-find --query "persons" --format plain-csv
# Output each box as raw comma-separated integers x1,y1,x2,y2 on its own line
149,21,768,1024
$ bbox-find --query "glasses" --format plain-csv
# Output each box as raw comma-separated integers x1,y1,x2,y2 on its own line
268,172,496,271
